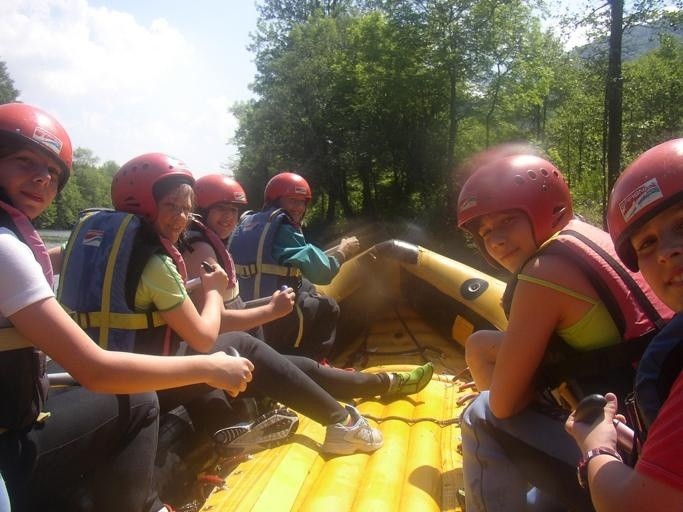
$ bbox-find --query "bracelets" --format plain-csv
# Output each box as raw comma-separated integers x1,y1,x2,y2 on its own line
570,449,623,493
335,249,347,261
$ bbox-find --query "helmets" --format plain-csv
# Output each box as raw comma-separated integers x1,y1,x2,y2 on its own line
111,153,194,224
264,172,312,202
195,175,249,209
607,138,683,272
458,155,573,270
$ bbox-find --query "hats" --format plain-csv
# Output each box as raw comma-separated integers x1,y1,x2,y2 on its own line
0,103,72,192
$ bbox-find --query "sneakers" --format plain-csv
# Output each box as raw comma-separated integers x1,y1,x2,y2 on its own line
381,362,434,397
212,409,299,457
323,404,384,454
320,358,355,372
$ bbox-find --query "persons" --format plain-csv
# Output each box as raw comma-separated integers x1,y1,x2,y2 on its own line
226,166,370,369
0,102,255,512
445,139,683,511
40,154,388,455
175,169,437,403
453,151,672,484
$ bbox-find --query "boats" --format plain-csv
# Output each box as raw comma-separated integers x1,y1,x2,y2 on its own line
148,236,515,512
